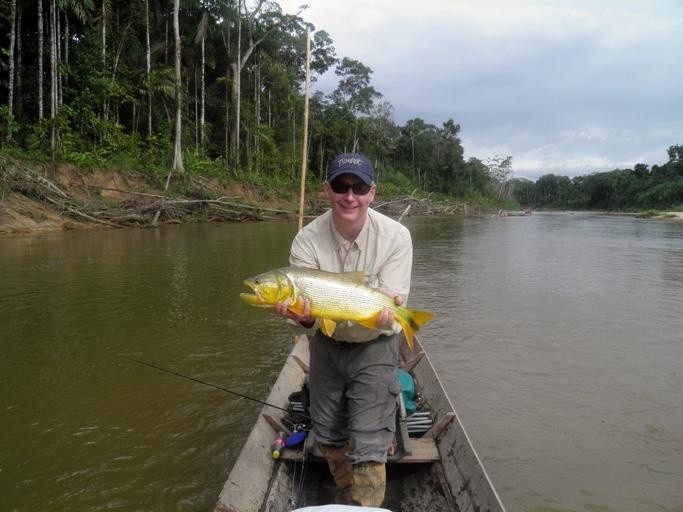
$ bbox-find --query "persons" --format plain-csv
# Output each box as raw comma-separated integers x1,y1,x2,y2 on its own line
277,152,413,508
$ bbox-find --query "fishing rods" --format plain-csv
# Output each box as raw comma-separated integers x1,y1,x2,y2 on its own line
116,354,311,422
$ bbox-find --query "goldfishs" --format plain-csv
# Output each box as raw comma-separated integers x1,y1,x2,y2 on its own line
239,265,436,351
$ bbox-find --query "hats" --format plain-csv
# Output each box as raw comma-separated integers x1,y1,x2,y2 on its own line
327,153,374,186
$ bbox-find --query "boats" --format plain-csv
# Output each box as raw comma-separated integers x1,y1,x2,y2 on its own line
210,320,505,512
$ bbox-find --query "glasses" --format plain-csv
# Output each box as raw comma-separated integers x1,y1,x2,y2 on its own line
330,180,370,195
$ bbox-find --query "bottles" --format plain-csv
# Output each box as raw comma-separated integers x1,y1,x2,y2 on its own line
270,430,285,460
